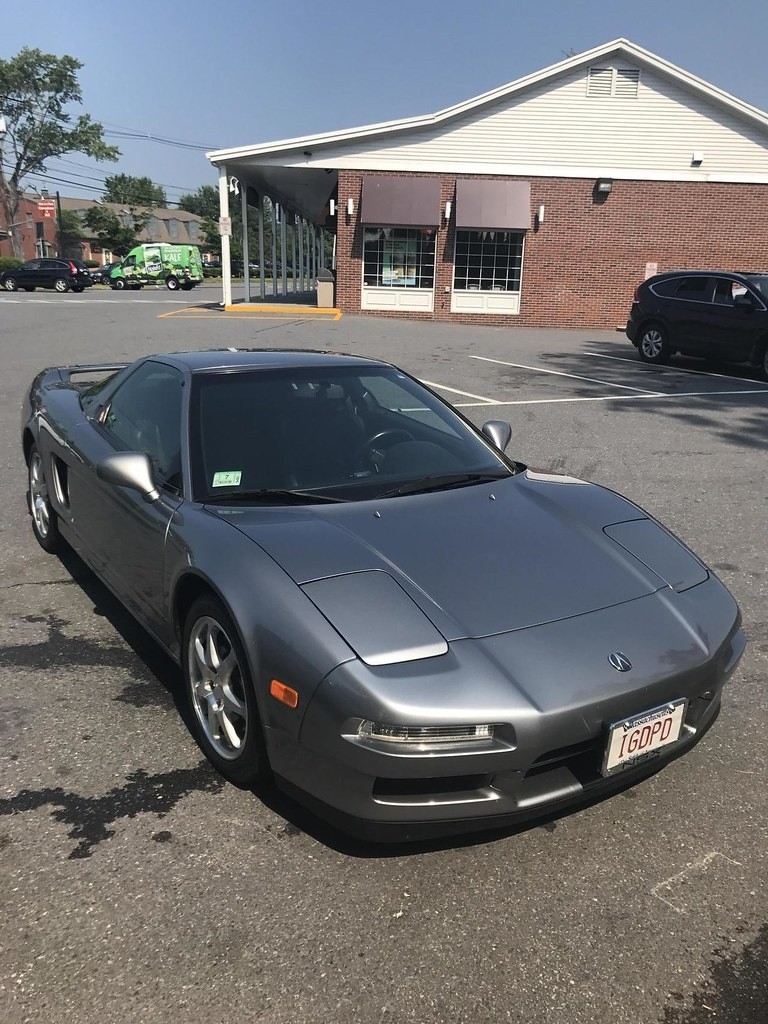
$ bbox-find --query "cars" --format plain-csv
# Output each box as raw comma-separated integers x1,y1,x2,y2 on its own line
89,262,121,285
202,259,273,270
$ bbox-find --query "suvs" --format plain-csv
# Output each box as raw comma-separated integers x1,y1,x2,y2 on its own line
0,257,94,293
626,269,768,382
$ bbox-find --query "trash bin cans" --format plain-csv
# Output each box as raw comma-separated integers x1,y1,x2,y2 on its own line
316,267,335,307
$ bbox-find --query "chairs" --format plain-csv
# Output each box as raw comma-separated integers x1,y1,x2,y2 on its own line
278,395,355,477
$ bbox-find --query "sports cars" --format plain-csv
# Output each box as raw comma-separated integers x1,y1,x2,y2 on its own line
19,346,746,840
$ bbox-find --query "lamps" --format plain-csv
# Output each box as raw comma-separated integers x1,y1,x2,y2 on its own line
228,176,240,196
347,198,353,215
445,201,452,219
538,206,544,221
330,199,335,217
596,178,612,192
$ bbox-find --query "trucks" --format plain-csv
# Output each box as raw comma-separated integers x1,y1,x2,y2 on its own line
110,242,204,290
